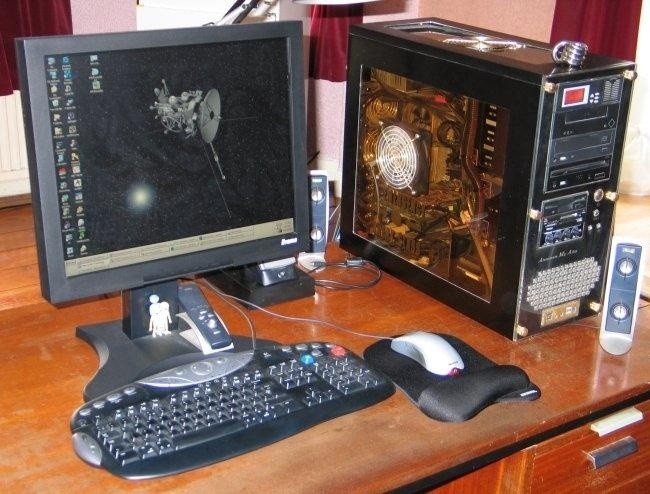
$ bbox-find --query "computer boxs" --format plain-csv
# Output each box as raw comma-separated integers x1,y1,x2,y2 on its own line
339,17,637,340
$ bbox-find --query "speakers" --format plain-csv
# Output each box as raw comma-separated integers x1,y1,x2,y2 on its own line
299,170,331,273
598,234,644,357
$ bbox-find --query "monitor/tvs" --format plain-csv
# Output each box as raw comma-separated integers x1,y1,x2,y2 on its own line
16,20,312,402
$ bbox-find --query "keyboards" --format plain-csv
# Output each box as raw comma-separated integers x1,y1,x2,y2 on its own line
69,341,394,483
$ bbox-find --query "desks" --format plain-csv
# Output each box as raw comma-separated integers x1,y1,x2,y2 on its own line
0,195,650,492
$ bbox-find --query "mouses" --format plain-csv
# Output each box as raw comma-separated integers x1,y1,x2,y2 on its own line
391,330,465,377
